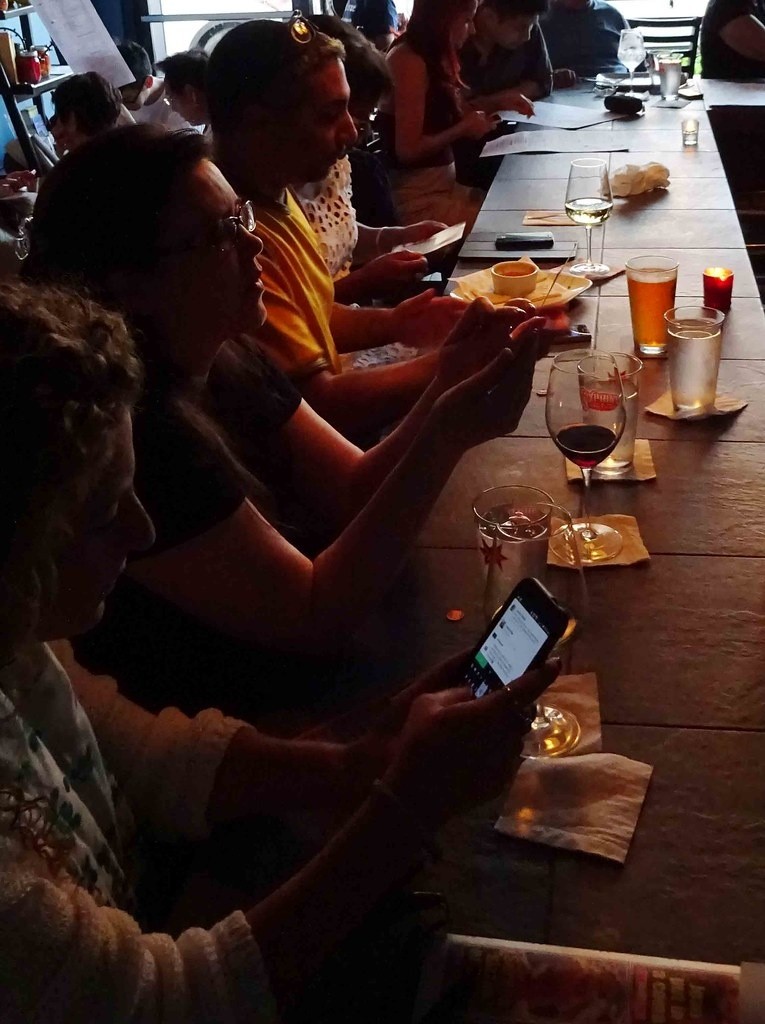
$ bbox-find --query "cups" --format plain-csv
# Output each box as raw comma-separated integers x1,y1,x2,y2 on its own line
471,484,554,625
681,121,699,146
578,349,645,480
624,254,680,355
663,304,725,413
659,56,682,101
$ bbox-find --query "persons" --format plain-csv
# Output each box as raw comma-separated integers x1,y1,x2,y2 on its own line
349,0,662,235
291,15,453,303
0,277,563,1024
207,20,537,451
23,125,551,731
0,39,209,282
701,0,765,187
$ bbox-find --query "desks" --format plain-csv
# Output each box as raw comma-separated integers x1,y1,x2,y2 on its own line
313,74,765,1024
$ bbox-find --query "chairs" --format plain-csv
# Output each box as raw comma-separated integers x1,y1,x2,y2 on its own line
627,17,702,77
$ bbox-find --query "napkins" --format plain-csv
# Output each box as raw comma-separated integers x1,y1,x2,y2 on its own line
644,389,748,419
520,670,602,758
523,210,602,227
565,439,657,483
491,749,654,864
547,513,650,567
448,257,561,304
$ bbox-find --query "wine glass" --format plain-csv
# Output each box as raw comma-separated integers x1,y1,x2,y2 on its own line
483,501,589,761
616,29,648,101
565,162,614,279
544,347,624,565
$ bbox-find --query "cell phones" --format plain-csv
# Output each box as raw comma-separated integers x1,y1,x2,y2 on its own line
540,324,592,346
495,232,554,251
452,575,570,699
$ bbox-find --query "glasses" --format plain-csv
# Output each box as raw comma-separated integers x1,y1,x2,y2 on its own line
121,75,149,108
262,8,319,82
144,201,258,274
45,113,60,133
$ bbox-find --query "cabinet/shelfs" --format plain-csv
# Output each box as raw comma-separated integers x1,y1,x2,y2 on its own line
0,0,74,178
